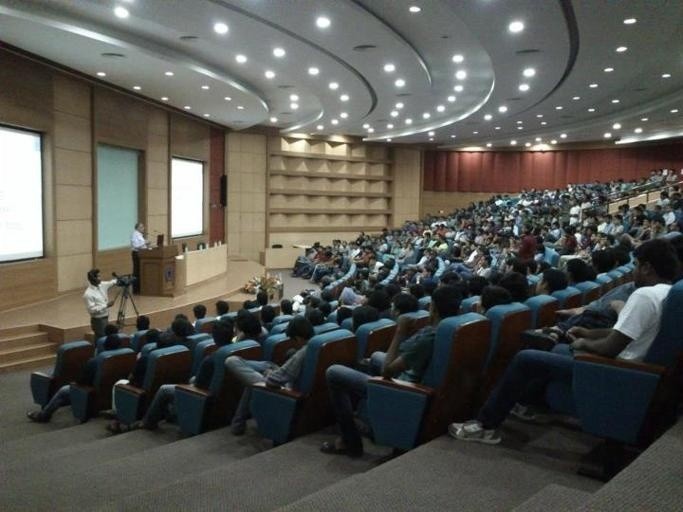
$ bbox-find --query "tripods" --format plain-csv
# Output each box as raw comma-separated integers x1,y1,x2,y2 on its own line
113,288,141,329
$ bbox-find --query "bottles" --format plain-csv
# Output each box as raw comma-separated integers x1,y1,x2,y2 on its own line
199,243,209,250
184,246,189,252
214,240,221,247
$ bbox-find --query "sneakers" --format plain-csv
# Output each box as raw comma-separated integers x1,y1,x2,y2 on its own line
447,417,505,446
318,434,365,457
508,402,538,421
25,407,157,434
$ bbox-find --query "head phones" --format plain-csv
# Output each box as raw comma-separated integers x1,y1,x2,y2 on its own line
89,269,97,284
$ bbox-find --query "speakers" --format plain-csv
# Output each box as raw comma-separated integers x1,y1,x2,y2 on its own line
220,175,228,207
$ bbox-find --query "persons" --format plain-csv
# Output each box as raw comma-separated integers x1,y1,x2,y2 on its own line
108,320,234,433
28,333,121,424
28,168,683,361
224,314,316,436
130,221,152,295
319,283,461,455
104,331,177,418
83,269,121,357
448,239,680,444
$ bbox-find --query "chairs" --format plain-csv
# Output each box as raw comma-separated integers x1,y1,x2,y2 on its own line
30,183,683,483
